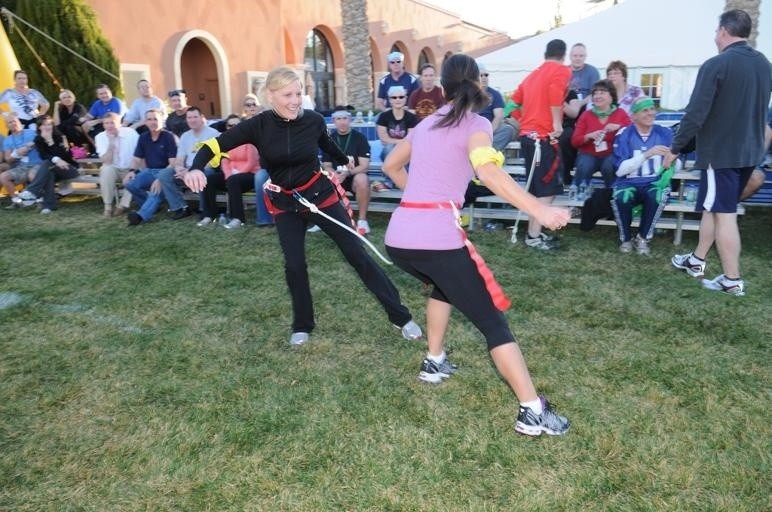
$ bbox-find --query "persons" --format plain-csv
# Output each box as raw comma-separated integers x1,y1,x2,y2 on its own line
658,7,771,295
381,52,573,438
459,39,771,255
184,63,424,348
1,71,274,229
307,51,448,234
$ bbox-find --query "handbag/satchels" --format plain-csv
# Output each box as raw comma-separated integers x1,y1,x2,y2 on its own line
69,146,89,159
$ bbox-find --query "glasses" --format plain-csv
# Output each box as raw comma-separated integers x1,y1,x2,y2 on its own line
389,61,402,64
388,96,405,99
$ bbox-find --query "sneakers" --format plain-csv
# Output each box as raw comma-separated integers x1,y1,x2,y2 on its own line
703,275,744,298
17,192,50,214
103,208,124,217
524,231,559,251
393,320,423,338
173,206,192,220
307,224,321,233
127,212,142,225
224,218,240,229
514,397,568,436
672,252,707,277
357,219,370,234
619,233,650,255
419,357,458,382
197,217,212,227
290,332,308,343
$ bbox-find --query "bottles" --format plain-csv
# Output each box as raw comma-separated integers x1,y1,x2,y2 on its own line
568,179,577,201
685,184,697,206
579,179,587,199
367,111,374,125
593,131,606,147
353,111,365,124
482,222,501,232
219,213,226,224
